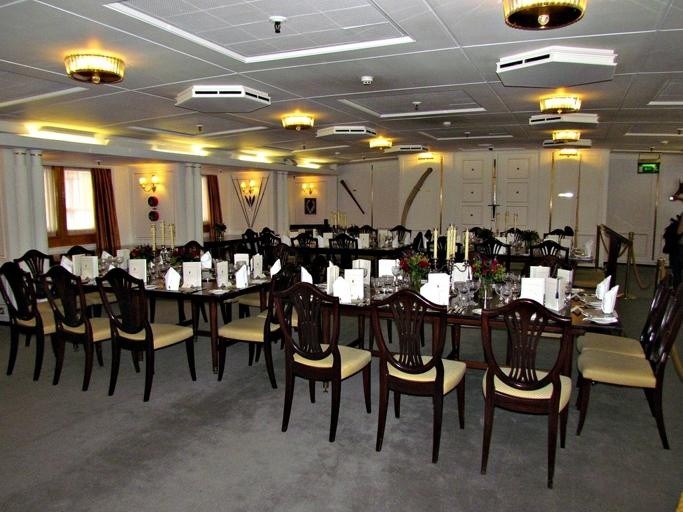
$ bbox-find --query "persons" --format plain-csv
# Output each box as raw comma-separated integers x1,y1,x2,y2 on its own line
663,210,682,287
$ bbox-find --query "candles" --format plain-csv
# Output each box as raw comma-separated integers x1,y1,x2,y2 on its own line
330,210,346,230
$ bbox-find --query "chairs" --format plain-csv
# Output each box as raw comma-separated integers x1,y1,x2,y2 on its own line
272,281,373,443
577,273,677,360
574,284,682,451
0,261,64,382
217,262,298,389
1,225,683,348
95,267,197,402
40,265,121,392
369,288,467,465
480,297,573,488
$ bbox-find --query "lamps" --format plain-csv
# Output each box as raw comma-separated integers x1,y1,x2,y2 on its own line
539,95,582,114
369,135,393,151
502,0,588,30
240,179,256,195
559,148,578,157
138,175,160,192
64,53,126,84
281,115,315,132
552,130,581,143
302,183,315,195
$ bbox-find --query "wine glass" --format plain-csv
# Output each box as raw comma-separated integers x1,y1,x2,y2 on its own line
373,267,622,325
96,250,245,289
